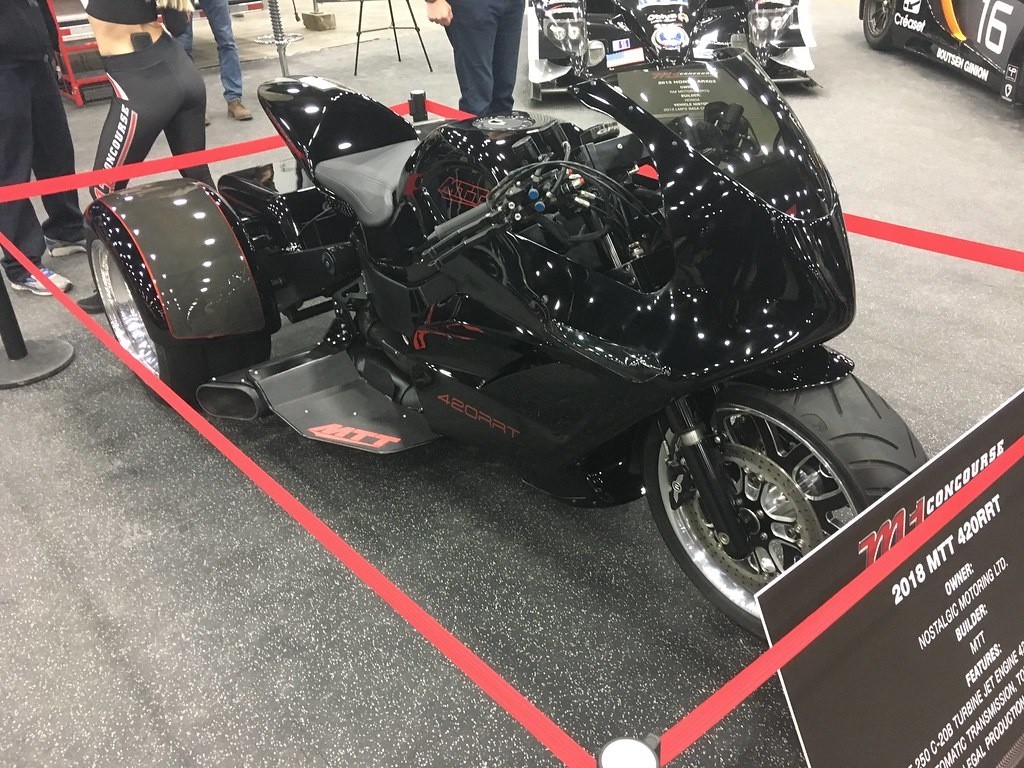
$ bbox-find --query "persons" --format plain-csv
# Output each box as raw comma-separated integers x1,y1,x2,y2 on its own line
78,0,217,313
424,0,526,115
172,0,253,125
0,0,89,296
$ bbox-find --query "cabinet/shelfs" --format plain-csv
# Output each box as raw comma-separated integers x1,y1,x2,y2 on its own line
47,0,109,105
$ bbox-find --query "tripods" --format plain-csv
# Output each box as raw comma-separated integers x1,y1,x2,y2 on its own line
354,0,434,76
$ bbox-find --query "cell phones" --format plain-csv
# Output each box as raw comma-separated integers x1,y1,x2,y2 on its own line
130,32,154,51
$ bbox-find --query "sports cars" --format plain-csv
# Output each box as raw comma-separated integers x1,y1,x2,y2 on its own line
526,0,824,101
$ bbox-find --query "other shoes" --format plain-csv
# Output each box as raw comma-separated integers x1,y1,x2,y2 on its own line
77,290,106,314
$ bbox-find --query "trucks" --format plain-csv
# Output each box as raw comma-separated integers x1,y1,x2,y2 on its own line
49,0,266,40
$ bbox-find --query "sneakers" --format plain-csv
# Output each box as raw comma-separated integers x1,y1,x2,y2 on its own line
204,111,211,125
11,267,74,295
228,99,253,120
42,235,89,257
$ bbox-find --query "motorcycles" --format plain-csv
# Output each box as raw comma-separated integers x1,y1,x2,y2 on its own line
82,47,927,645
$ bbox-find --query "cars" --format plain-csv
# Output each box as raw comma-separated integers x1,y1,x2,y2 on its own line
857,1,1024,107
